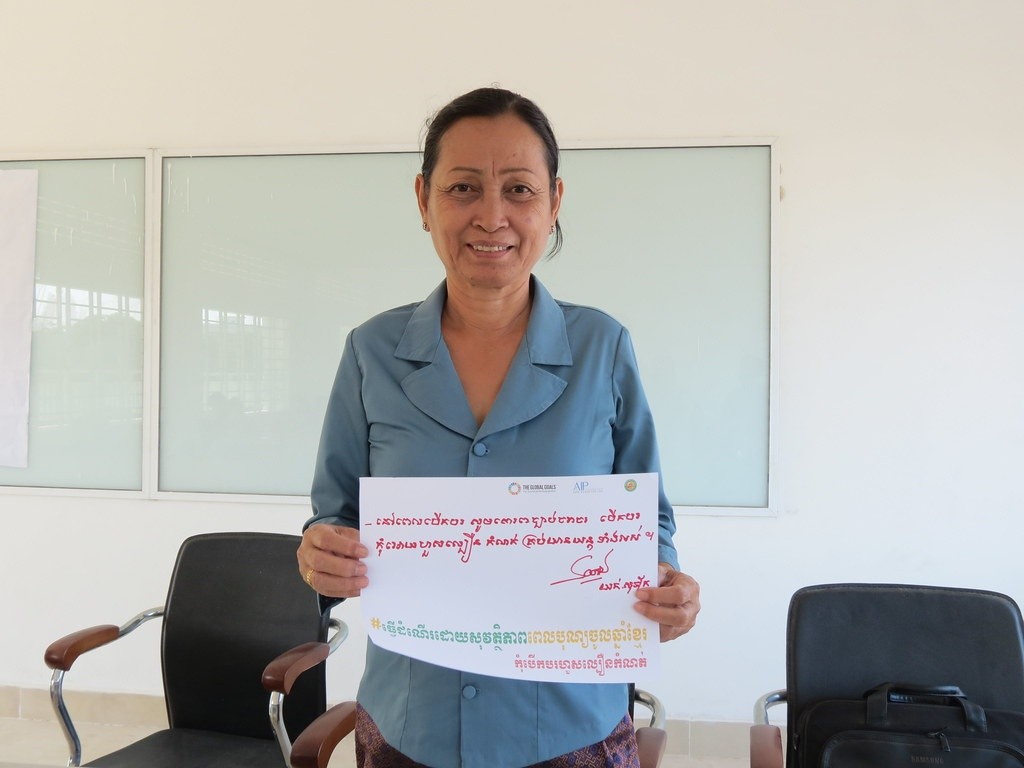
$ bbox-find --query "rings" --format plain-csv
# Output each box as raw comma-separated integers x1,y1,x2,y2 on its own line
306,569,314,586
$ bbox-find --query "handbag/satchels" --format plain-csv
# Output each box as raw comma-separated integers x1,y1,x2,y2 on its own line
791,678,1024,768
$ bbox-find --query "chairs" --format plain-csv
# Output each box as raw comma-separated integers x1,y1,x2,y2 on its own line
41,531,351,768
290,687,668,768
748,582,1024,768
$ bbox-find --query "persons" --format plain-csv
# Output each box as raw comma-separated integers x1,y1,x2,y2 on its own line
296,86,702,768
